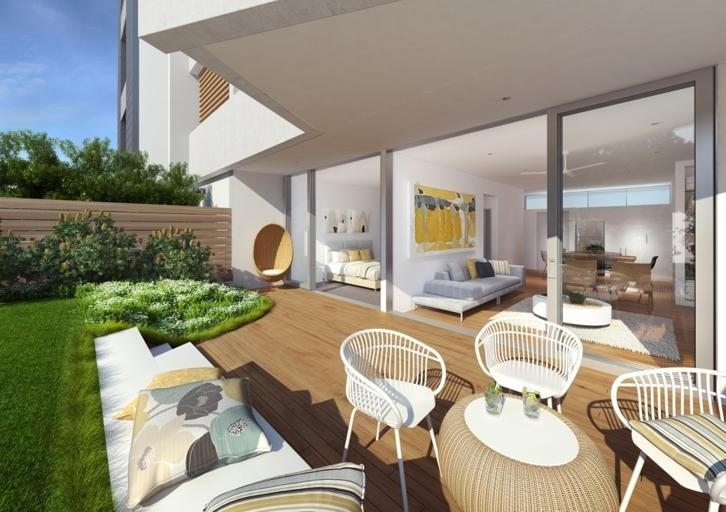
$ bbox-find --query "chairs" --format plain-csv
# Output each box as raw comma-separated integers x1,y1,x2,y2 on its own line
252,223,293,282
611,367,726,512
532,244,658,305
340,328,446,512
475,316,583,414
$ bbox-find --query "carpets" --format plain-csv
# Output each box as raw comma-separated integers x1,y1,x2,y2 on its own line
490,298,681,361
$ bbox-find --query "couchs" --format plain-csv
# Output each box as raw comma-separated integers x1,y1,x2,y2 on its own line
412,257,526,322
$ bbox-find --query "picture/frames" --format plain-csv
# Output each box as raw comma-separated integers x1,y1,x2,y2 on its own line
407,182,477,259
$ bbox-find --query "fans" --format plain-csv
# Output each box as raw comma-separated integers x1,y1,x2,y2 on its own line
520,150,603,178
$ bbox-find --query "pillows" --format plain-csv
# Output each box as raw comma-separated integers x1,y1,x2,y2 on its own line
114,367,225,420
333,249,372,263
629,414,726,483
446,259,510,281
205,462,366,512
127,378,272,510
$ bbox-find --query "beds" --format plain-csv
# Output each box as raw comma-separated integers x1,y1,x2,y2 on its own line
323,239,380,292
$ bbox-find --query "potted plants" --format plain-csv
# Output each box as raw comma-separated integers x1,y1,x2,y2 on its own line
568,292,585,304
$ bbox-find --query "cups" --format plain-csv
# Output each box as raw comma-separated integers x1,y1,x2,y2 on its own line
483,383,506,416
522,386,541,422
